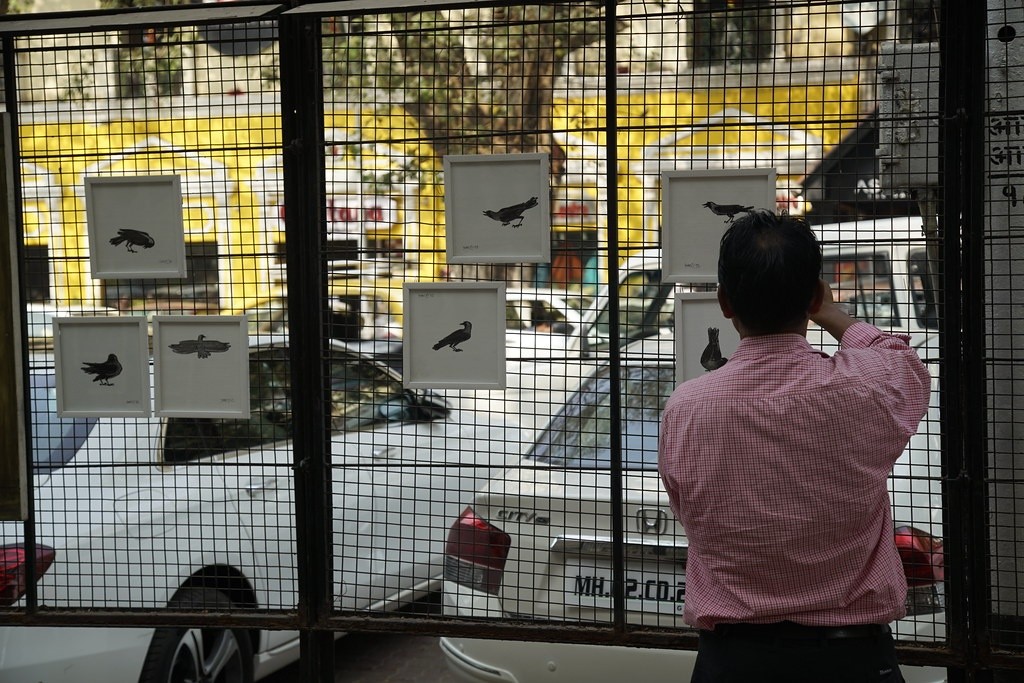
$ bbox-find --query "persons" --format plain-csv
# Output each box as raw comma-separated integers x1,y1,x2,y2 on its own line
522,308,555,333
662,210,932,681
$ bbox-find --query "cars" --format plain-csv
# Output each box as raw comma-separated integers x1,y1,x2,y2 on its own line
570,211,941,370
436,330,939,681
503,287,592,359
211,281,409,354
0,332,531,683
